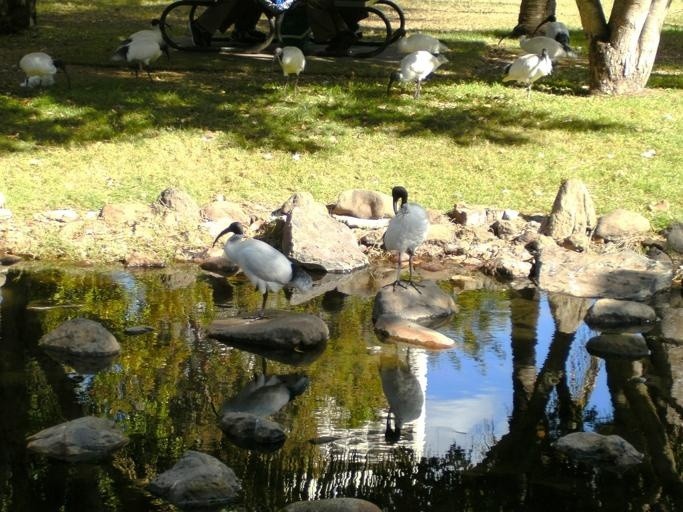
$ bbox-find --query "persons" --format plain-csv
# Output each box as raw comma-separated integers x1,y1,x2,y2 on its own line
304,0,368,51
189,0,266,48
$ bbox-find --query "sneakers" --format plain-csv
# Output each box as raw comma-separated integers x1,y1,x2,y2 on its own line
325,30,359,52
189,20,211,48
232,28,266,43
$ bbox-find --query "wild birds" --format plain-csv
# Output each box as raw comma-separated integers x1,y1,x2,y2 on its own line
383,28,453,98
376,343,424,445
379,185,430,295
214,355,310,422
274,46,306,89
17,51,72,90
498,14,575,94
212,222,313,321
110,19,173,83
